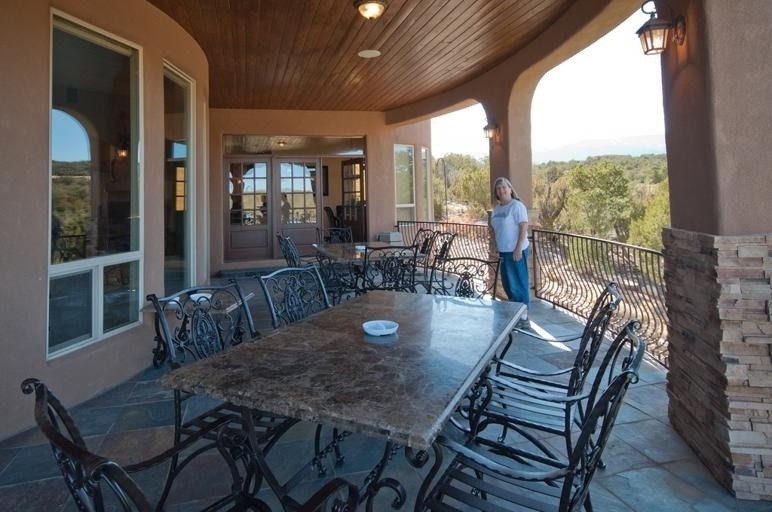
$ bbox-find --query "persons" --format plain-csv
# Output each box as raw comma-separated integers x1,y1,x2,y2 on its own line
489,176,532,330
231,194,290,225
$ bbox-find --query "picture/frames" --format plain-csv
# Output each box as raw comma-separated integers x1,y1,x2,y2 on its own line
307,166,328,196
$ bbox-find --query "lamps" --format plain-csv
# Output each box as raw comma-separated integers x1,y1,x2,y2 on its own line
635,0,687,55
354,1,388,20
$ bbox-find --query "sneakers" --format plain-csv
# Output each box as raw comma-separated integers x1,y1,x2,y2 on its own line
515,319,530,330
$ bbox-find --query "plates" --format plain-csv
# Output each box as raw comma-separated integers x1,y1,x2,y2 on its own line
362,320,399,337
